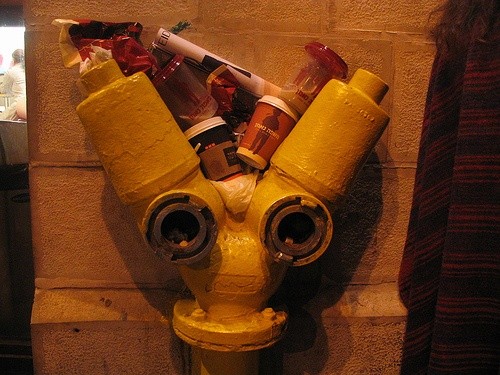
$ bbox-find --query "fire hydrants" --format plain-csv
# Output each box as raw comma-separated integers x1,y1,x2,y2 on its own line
73,59,392,375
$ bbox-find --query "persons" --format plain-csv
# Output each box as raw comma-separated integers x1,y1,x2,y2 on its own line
397,1,499,374
0,48,25,124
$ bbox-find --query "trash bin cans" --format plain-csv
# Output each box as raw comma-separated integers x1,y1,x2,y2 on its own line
0,118,35,340
